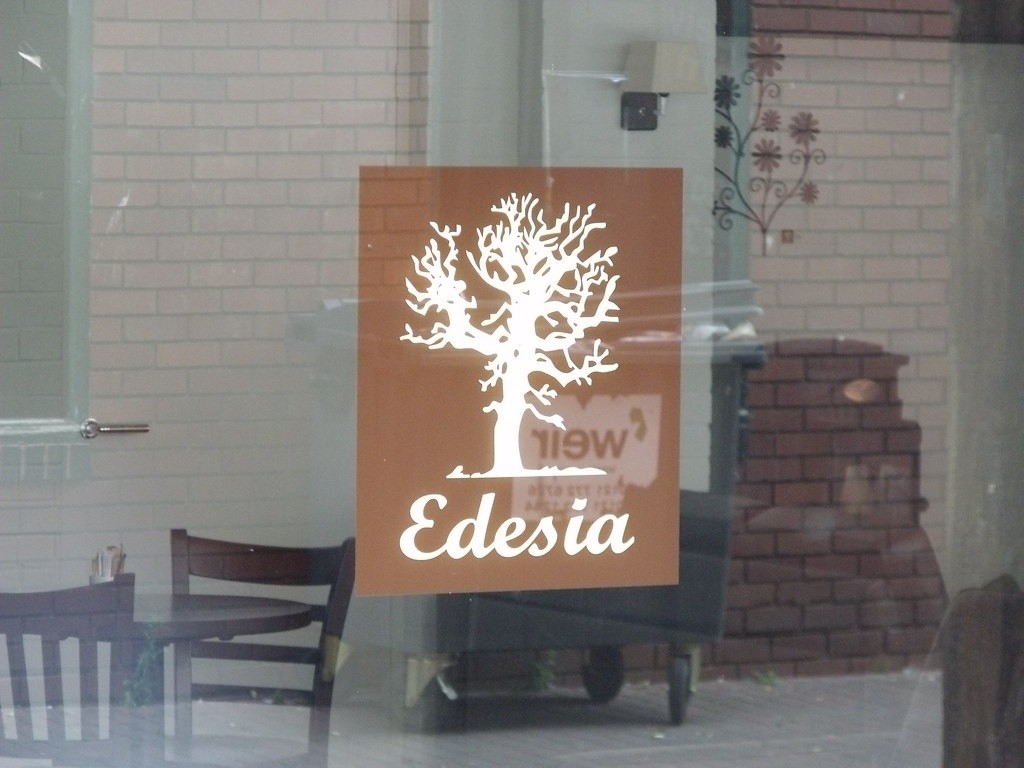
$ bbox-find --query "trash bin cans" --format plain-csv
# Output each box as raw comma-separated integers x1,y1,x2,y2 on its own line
423,324,768,726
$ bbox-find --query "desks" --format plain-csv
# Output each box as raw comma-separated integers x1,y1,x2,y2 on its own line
35,593,311,766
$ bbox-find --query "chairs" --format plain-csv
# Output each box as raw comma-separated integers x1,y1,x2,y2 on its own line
0,531,359,766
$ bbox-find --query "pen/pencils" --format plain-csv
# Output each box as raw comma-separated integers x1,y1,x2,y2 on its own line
91,545,126,577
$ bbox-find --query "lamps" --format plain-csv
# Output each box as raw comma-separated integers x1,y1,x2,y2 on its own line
620,40,708,130
